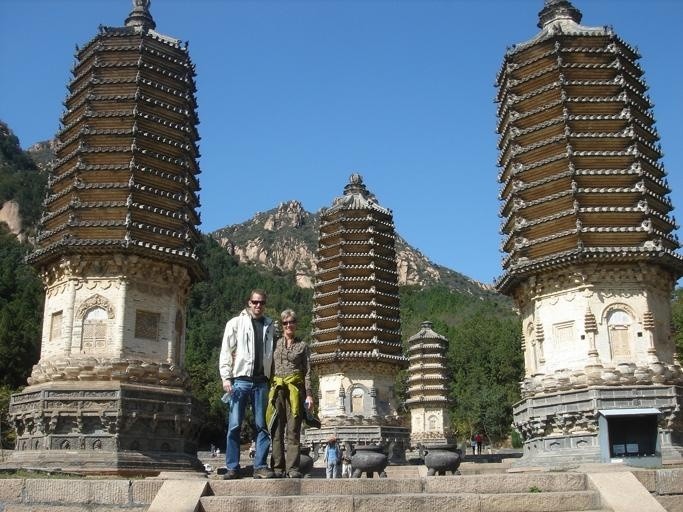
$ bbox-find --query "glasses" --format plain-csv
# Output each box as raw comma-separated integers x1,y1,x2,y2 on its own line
249,298,266,305
283,319,296,325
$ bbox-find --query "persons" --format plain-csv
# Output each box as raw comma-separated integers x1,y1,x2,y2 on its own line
217,289,278,480
342,443,354,478
209,443,215,457
248,444,252,458
323,437,343,478
251,439,256,458
470,434,476,456
216,448,221,457
267,309,315,478
476,433,483,456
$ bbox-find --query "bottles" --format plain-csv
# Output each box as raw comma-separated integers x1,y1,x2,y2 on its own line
302,399,314,422
219,382,238,403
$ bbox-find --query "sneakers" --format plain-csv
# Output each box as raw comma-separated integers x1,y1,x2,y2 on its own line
252,466,284,479
285,470,303,478
222,469,243,480
